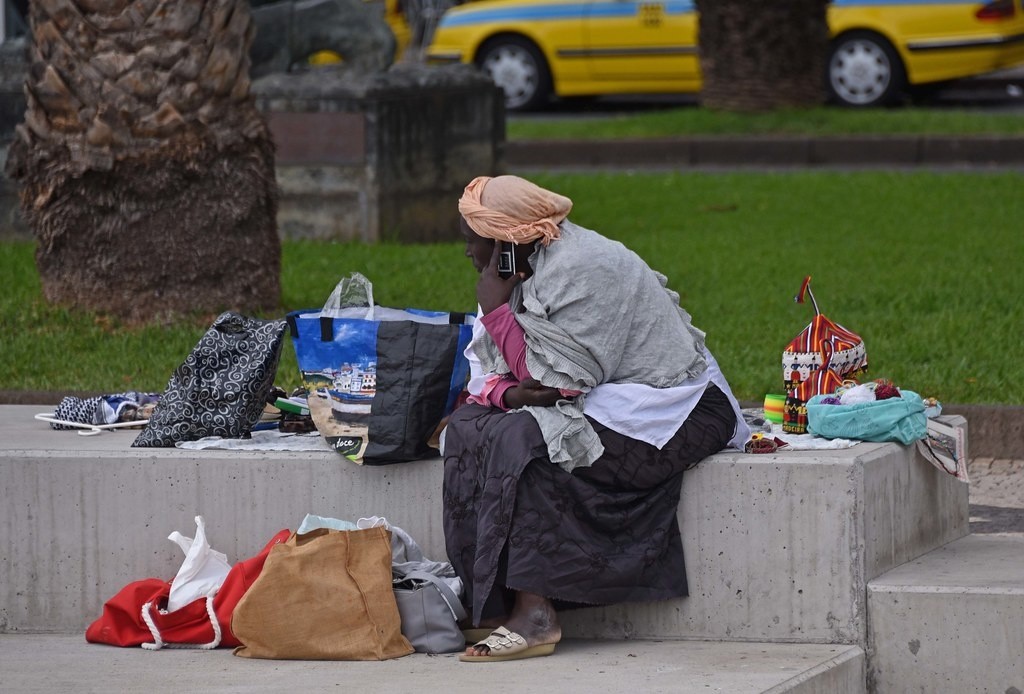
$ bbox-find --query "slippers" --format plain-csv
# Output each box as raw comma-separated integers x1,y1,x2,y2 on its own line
459,621,557,663
458,618,492,642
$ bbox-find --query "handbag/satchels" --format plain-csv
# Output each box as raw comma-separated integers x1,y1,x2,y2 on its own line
805,391,929,444
84,513,467,662
287,276,473,465
131,309,290,449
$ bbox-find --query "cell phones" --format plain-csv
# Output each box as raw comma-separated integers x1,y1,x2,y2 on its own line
498,240,516,280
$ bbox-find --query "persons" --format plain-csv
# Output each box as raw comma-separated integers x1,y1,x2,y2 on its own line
438,174,748,663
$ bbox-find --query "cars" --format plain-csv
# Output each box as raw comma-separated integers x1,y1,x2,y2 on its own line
305,0,1024,119
